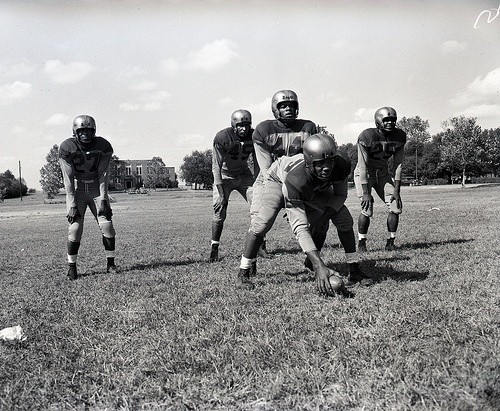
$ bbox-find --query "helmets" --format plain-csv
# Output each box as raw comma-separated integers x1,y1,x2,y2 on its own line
375,107,397,130
73,115,96,138
303,134,336,178
272,90,298,120
231,110,251,134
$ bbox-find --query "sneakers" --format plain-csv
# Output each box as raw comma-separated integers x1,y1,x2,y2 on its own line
69,268,77,280
107,265,118,275
358,238,367,252
250,260,257,277
238,268,255,291
211,244,219,262
385,237,399,251
350,262,373,286
258,241,272,258
305,250,324,274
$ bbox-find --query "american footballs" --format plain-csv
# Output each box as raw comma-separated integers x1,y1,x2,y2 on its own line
328,273,346,294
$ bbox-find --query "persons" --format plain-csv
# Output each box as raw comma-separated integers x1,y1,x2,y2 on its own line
252,90,330,270
59,114,117,279
238,132,379,296
210,110,274,261
353,107,407,250
